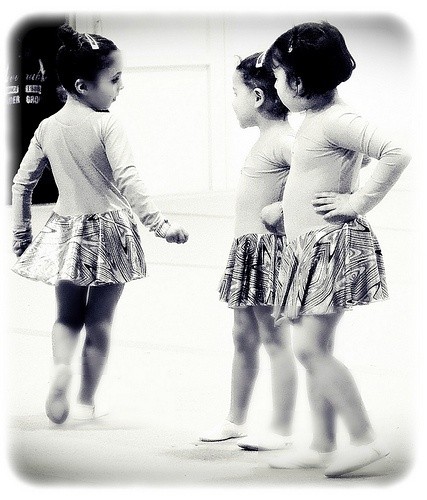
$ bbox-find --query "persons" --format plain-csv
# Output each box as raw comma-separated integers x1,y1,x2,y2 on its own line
198,50,299,452
9,33,189,424
265,21,413,478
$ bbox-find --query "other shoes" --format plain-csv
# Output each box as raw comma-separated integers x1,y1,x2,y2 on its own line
237,430,291,451
268,444,337,470
325,437,391,477
199,418,248,442
46,364,73,425
75,403,113,420
